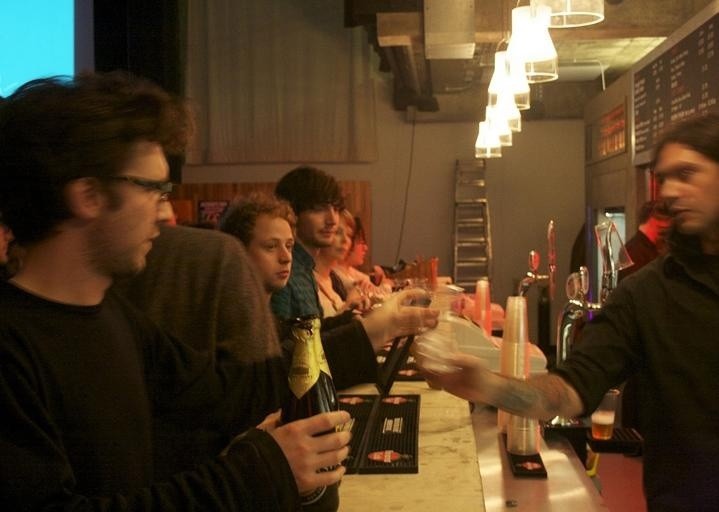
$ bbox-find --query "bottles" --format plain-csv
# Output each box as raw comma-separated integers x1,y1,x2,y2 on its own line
281,313,341,512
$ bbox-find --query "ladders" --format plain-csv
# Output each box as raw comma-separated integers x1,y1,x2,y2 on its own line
451,157,495,297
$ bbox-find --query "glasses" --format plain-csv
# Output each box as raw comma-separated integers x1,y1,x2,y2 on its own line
90,171,175,199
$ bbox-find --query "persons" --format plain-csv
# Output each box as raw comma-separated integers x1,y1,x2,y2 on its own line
617,199,669,287
430,115,719,512
1,73,439,512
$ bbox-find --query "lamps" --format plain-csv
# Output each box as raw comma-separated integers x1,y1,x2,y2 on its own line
485,94,522,133
487,37,530,113
474,122,502,160
506,0,559,86
485,117,513,146
530,1,605,28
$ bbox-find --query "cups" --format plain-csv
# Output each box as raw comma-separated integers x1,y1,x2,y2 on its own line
499,296,539,455
473,280,492,338
592,389,620,439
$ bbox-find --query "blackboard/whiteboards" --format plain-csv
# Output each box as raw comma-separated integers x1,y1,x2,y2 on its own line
631,12,719,167
